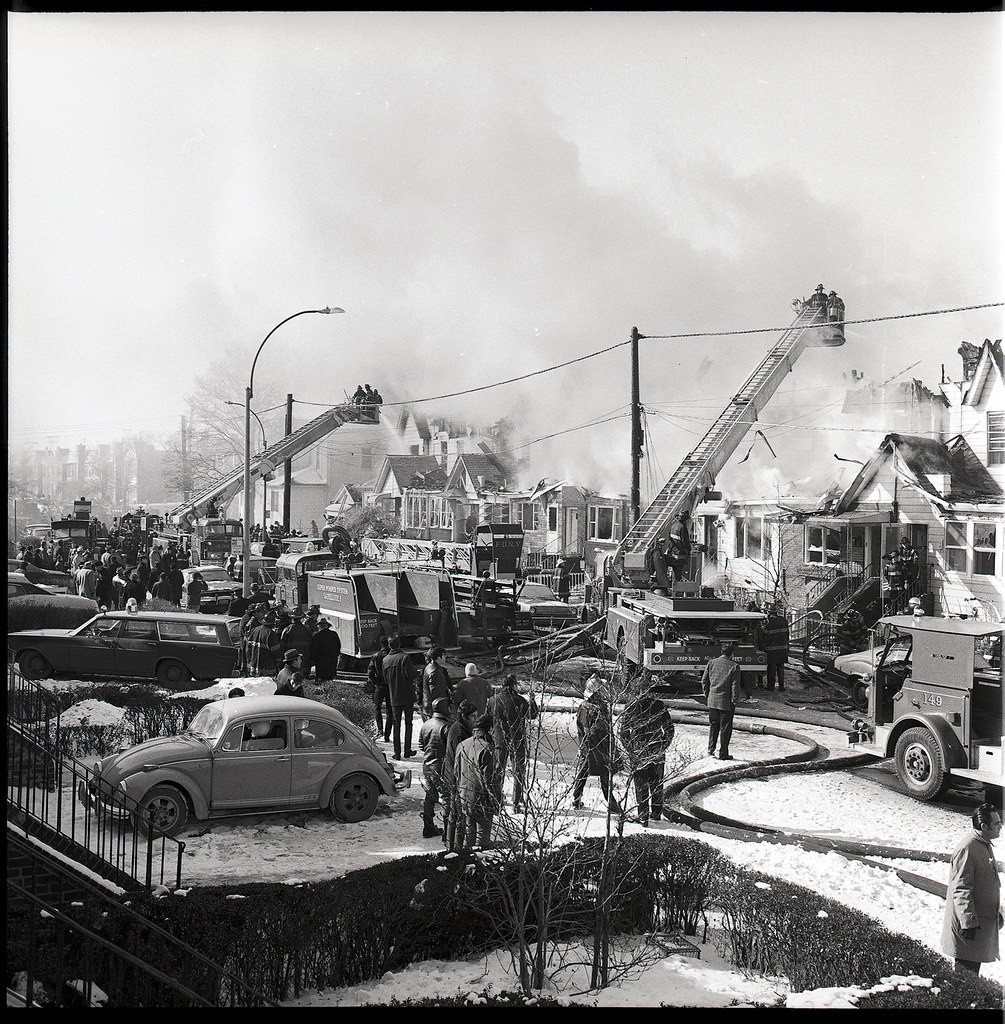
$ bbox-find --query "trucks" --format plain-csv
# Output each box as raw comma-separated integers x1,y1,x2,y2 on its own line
273,548,459,673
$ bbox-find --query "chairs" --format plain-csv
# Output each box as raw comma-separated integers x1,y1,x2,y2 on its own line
271,725,282,738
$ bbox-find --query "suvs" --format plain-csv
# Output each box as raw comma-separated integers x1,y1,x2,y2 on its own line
6,597,242,690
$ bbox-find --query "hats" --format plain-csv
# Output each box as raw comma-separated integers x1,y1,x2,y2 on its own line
95,561,104,565
556,559,566,567
282,648,302,662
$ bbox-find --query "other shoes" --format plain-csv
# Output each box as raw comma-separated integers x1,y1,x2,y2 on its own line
635,818,648,827
609,807,624,813
423,824,443,838
573,802,584,810
726,755,734,760
377,731,417,760
514,807,523,814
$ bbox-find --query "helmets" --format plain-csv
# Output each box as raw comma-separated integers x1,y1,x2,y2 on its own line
316,618,332,628
271,600,285,609
844,536,910,621
258,613,276,625
583,673,608,701
763,603,777,613
287,607,306,618
674,510,691,521
306,605,321,615
251,604,266,611
244,604,258,612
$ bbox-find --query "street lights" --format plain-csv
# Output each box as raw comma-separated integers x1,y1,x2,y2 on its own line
224,400,267,543
241,305,346,602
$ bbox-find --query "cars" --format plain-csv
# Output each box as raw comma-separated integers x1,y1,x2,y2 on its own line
85,697,413,841
497,580,577,632
25,524,52,540
8,559,75,596
249,556,276,596
250,542,265,556
181,568,243,609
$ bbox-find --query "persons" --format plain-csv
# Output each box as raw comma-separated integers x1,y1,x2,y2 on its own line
552,559,571,603
806,283,844,316
227,554,243,583
245,721,270,749
310,520,319,538
940,803,1002,975
250,520,299,544
840,609,868,655
418,647,537,813
262,538,280,558
97,521,118,537
747,603,789,690
701,644,740,759
186,572,208,612
14,530,191,610
228,582,342,697
353,384,383,418
367,635,417,760
665,510,692,581
619,677,675,826
572,673,624,813
883,536,918,607
418,697,501,846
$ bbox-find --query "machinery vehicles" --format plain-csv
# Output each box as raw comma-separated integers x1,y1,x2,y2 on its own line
600,312,844,696
833,604,1005,811
150,396,382,569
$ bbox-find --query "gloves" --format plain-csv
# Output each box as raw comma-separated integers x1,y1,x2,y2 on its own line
959,928,975,940
